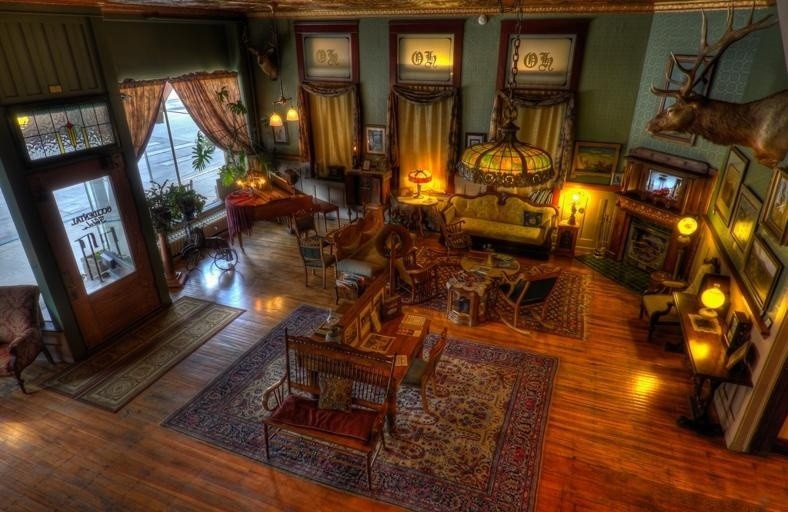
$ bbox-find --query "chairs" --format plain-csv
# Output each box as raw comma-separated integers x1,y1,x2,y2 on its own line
496,269,558,336
638,256,720,342
394,326,449,414
292,213,333,253
0,284,56,396
295,235,337,289
393,250,447,306
440,220,472,261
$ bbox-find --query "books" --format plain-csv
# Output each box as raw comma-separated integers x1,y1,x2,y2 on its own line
395,313,426,337
336,274,365,294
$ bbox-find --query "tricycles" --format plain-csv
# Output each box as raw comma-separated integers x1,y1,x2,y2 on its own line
181,222,239,271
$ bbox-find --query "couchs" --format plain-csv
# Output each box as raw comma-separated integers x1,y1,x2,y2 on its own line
438,190,559,259
333,208,416,282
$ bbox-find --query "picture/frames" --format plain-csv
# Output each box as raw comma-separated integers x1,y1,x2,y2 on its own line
464,131,488,149
650,53,716,148
363,124,385,156
271,122,289,144
728,185,763,254
362,158,371,170
724,340,752,371
760,167,788,246
570,141,621,177
714,145,750,228
742,232,783,311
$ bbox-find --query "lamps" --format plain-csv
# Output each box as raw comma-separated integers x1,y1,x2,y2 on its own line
668,216,698,295
453,0,557,189
267,2,300,127
699,285,726,318
235,170,266,192
568,194,579,225
407,168,433,199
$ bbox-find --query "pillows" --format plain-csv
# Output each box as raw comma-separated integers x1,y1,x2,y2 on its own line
523,209,543,228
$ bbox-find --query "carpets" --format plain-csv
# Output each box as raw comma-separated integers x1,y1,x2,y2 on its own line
37,294,247,415
405,244,596,343
159,302,560,511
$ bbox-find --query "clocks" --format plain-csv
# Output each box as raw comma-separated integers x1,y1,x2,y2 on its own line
722,310,750,356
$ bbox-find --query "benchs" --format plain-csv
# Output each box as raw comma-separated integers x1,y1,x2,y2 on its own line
310,198,341,232
261,325,396,492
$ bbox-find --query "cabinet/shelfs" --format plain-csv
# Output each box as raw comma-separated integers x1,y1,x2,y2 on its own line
345,165,393,224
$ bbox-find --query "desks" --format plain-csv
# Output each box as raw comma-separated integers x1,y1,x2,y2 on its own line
397,195,438,237
673,290,754,439
446,270,496,328
552,220,580,257
308,271,432,420
